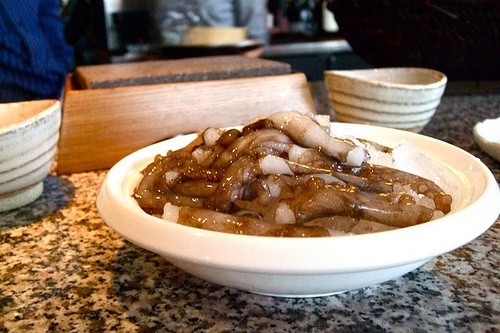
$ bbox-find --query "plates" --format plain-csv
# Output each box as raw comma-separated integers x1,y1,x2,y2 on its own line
473,117,500,160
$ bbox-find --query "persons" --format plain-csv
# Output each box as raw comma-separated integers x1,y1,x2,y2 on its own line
325,0,500,82
0,0,94,110
146,0,270,61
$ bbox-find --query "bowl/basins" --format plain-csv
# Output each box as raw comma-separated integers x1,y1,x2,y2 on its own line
94,121,500,299
0,99,62,212
322,68,448,133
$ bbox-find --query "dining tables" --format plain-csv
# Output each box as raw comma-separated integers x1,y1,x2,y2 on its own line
0,82,500,333
